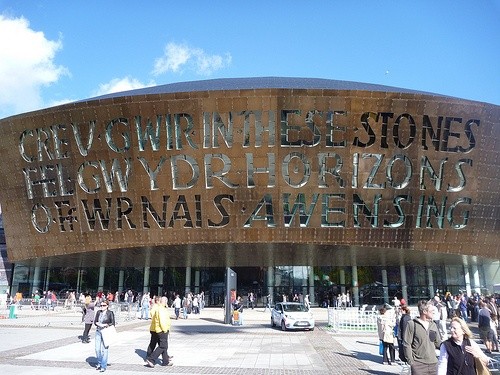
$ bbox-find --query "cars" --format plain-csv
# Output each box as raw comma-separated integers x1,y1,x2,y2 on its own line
270,302,316,331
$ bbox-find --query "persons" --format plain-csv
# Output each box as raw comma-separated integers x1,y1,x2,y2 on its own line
146,297,174,367
479,300,490,354
433,296,449,348
439,318,491,375
486,302,499,352
146,296,174,360
377,307,399,365
82,300,94,343
0,280,354,320
92,301,114,371
432,288,499,323
403,300,437,374
397,305,410,365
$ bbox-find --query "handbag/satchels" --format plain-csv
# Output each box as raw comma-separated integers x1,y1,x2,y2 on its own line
474,357,491,375
100,326,118,348
